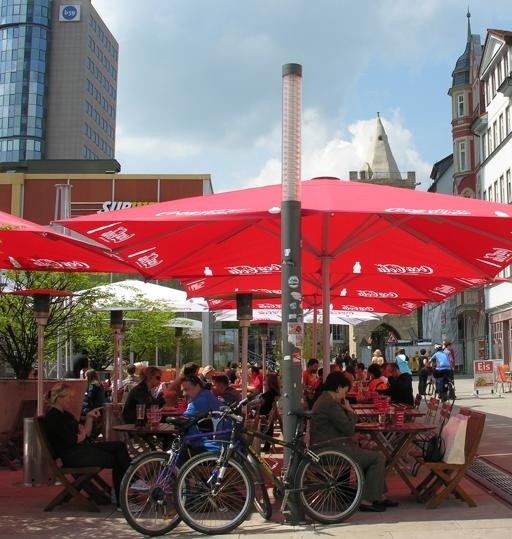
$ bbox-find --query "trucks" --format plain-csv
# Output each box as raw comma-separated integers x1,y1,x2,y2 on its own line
385,338,432,374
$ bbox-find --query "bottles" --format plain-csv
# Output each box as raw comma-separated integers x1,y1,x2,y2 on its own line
8,256,22,269
340,287,347,296
80,370,83,380
352,261,361,273
203,266,212,276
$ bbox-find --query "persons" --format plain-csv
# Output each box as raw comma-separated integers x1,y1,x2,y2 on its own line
35,340,455,514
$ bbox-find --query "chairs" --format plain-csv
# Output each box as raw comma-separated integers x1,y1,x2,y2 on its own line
0,400,37,471
83,391,282,500
496,363,512,394
37,414,112,511
348,390,486,511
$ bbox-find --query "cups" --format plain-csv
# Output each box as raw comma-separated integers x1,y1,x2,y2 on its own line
136,403,146,427
379,413,386,423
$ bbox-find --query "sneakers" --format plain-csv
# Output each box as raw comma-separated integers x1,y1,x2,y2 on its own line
117,502,144,512
130,479,152,492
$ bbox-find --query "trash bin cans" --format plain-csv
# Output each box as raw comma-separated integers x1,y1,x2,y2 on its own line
103,402,129,442
23,417,57,487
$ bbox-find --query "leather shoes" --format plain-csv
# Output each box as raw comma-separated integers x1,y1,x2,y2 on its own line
375,499,399,507
359,503,386,513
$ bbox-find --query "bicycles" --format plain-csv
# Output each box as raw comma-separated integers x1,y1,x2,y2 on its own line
182,392,364,535
420,368,456,410
266,354,279,375
122,392,273,538
